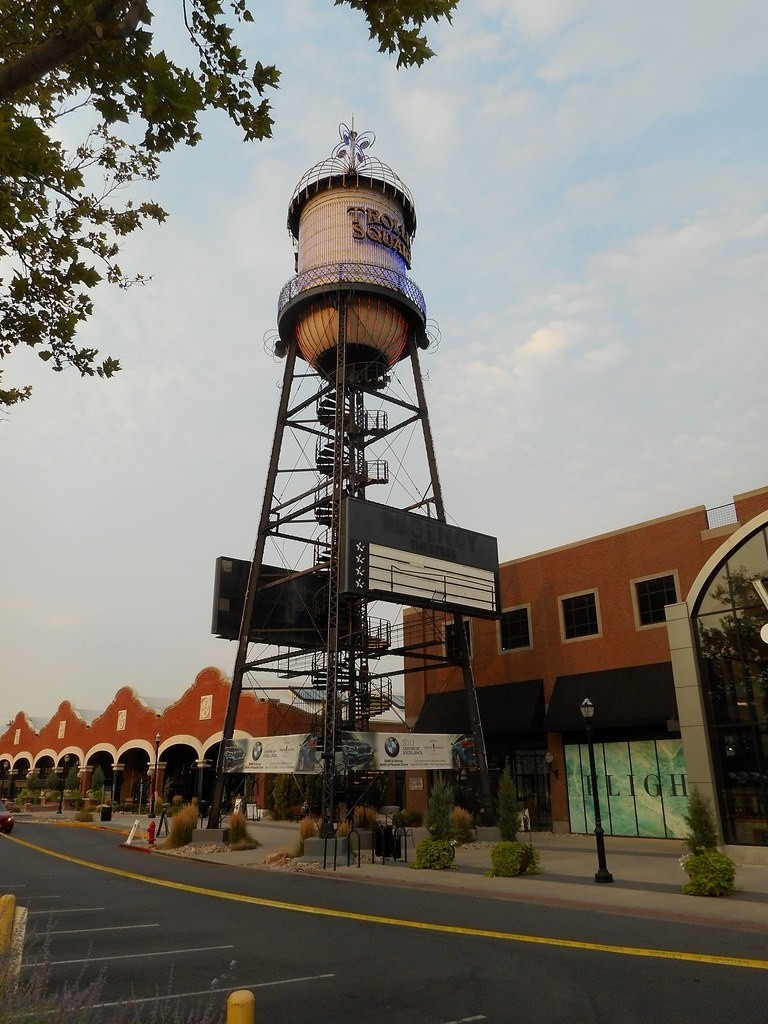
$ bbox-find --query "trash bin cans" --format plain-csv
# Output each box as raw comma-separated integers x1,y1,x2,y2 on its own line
101,806,113,821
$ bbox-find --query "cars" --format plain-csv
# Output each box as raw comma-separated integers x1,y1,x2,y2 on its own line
0,801,15,833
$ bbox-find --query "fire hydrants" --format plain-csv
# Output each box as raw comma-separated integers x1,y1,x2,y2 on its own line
147,821,156,845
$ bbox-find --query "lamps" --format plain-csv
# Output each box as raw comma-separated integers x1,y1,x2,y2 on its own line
544,751,559,779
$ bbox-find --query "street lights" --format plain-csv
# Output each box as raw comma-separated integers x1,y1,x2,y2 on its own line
57,755,69,813
580,698,612,882
0,761,8,801
148,732,161,818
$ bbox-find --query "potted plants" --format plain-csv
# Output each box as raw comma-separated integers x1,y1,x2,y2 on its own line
410,777,461,871
482,767,545,879
